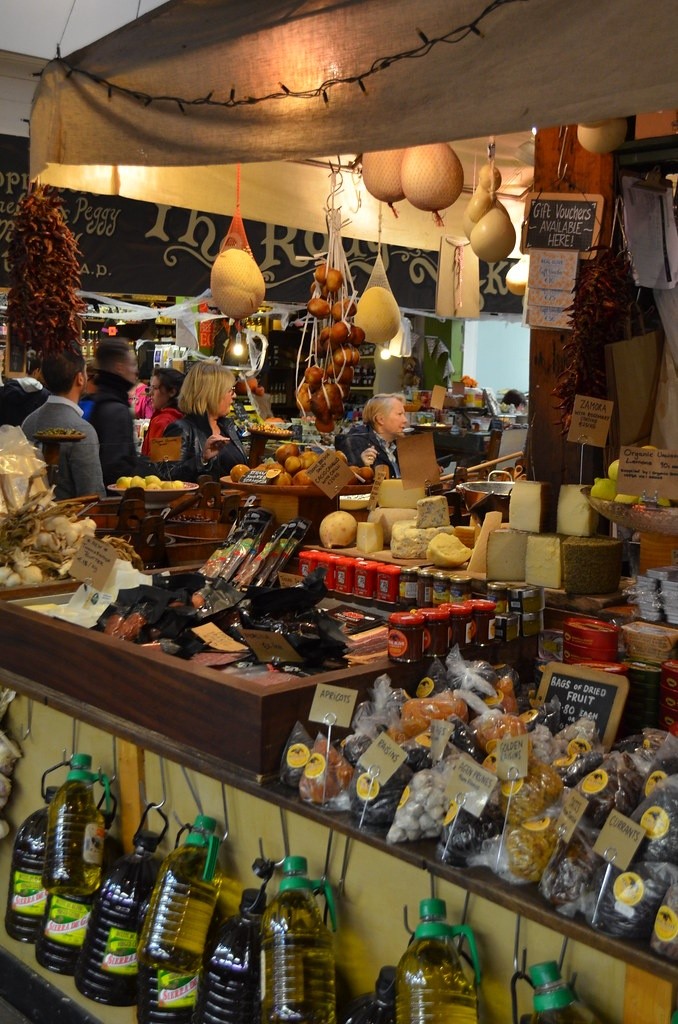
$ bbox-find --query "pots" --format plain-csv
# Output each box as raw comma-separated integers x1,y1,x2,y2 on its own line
458,470,515,526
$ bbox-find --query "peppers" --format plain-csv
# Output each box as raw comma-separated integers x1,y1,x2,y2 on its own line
546,244,642,439
4,184,89,363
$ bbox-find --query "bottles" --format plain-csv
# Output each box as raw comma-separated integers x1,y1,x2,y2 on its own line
136,960,197,1024
196,887,268,1024
246,317,262,334
35,891,95,975
136,814,227,976
259,855,338,1024
82,330,99,359
527,959,603,1024
4,785,60,944
41,753,102,897
74,803,170,1007
395,898,481,1024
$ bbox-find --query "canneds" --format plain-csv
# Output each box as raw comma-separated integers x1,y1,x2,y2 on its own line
388,566,545,664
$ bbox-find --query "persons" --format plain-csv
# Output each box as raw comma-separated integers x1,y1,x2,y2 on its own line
22,345,106,501
154,362,252,484
340,393,444,479
0,349,51,427
76,338,186,496
500,389,527,410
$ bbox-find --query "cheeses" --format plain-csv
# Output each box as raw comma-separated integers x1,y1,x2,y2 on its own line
319,481,627,595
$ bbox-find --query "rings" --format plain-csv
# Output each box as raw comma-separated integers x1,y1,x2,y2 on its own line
368,456,371,460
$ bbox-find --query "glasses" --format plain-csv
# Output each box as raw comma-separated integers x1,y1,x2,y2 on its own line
228,387,236,395
149,384,163,393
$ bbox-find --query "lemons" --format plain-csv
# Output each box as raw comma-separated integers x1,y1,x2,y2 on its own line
591,446,669,506
116,475,184,491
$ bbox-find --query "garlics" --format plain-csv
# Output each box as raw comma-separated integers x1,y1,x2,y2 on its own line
0,515,100,587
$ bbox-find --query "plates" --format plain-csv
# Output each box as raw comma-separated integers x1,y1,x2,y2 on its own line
34,431,86,440
106,480,200,509
